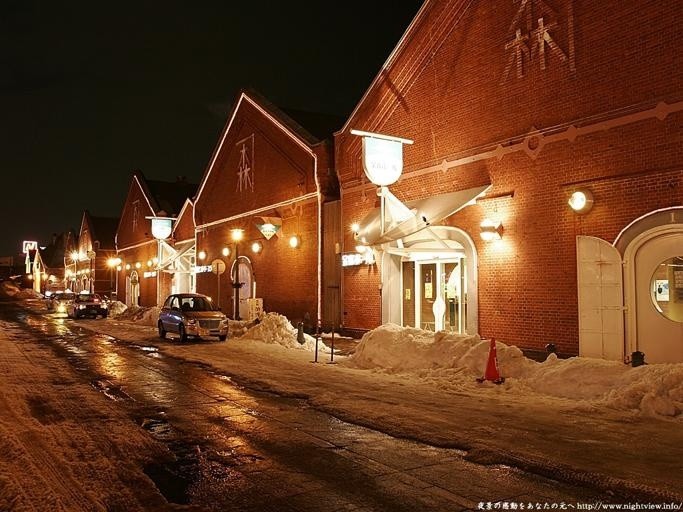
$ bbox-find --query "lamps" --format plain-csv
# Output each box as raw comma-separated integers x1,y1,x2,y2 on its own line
117,260,154,271
569,189,594,214
479,221,503,240
198,235,301,261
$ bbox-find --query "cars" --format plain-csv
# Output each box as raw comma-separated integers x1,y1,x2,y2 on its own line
66,293,108,320
157,293,229,342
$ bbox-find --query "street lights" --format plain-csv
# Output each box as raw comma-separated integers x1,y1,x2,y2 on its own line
229,227,245,321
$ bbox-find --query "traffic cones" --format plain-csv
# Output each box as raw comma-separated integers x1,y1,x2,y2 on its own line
475,336,505,385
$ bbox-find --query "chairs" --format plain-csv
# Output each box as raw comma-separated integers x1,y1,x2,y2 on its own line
182,303,193,311
